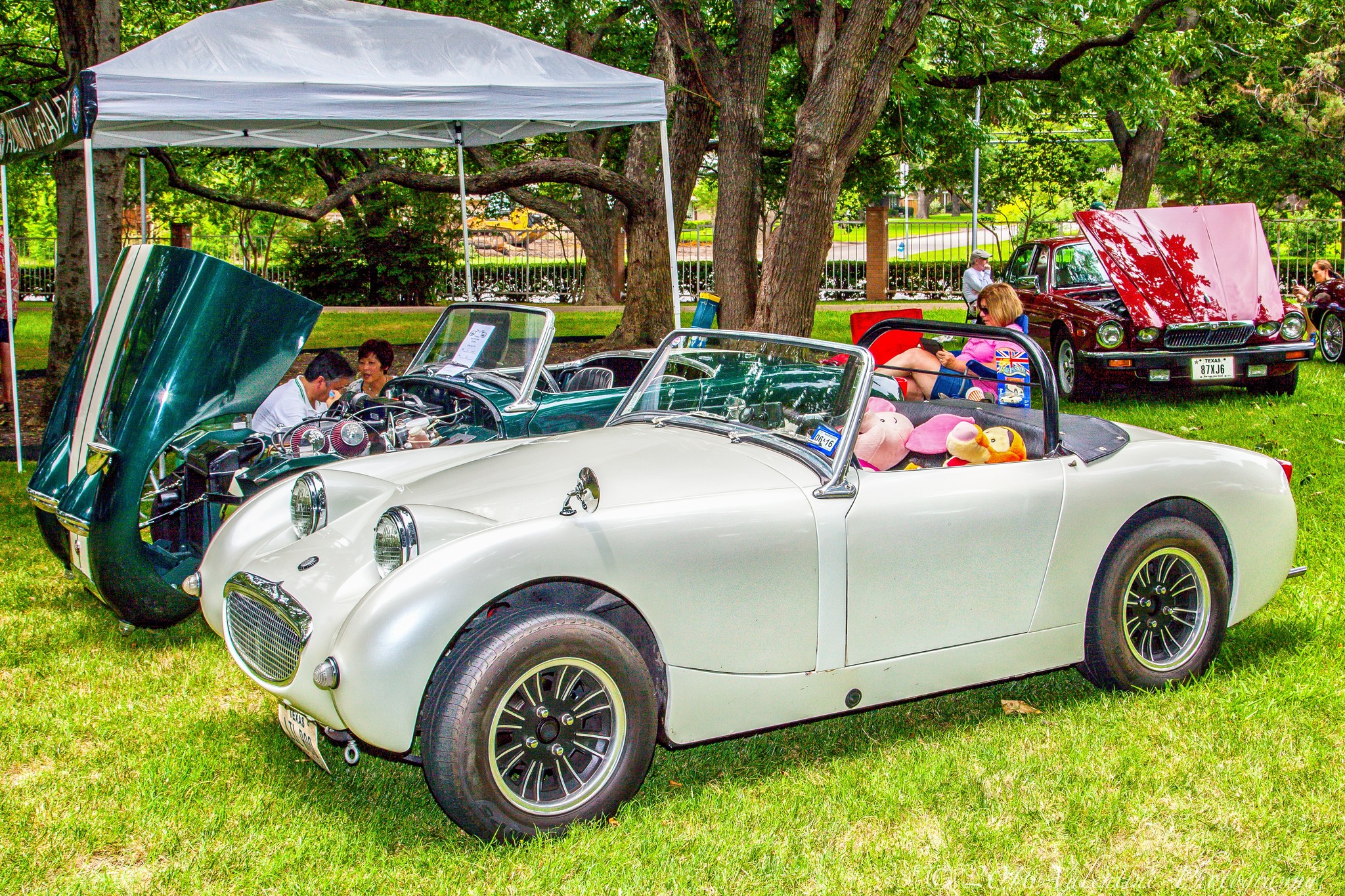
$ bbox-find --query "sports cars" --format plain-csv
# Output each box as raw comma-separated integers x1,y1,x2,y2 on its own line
25,246,759,625
174,324,1309,845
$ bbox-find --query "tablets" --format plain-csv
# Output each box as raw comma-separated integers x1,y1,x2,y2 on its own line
919,337,942,356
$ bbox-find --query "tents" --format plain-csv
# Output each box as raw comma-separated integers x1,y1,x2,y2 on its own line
1,0,681,472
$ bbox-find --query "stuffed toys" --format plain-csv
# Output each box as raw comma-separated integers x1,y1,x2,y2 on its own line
902,421,1028,474
814,396,977,474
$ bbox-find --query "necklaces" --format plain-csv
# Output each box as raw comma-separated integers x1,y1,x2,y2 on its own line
369,384,379,396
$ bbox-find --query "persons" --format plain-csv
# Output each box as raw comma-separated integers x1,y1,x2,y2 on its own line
1021,246,1058,285
1085,202,1107,212
343,338,401,397
961,250,997,315
1291,257,1345,347
871,283,1029,403
0,224,19,412
250,347,356,437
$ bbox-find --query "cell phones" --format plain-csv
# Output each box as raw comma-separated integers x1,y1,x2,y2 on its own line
1292,280,1297,288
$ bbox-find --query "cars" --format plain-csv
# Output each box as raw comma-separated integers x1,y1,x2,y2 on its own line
1302,281,1345,364
988,201,1321,396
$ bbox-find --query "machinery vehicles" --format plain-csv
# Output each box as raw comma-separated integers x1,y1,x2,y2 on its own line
428,197,551,245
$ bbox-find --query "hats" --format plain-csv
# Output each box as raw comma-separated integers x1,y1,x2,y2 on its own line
970,249,993,261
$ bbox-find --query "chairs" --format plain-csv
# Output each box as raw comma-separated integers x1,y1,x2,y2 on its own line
562,368,615,393
437,311,512,371
826,308,926,376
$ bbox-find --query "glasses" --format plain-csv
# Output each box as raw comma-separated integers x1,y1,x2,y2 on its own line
978,306,990,315
